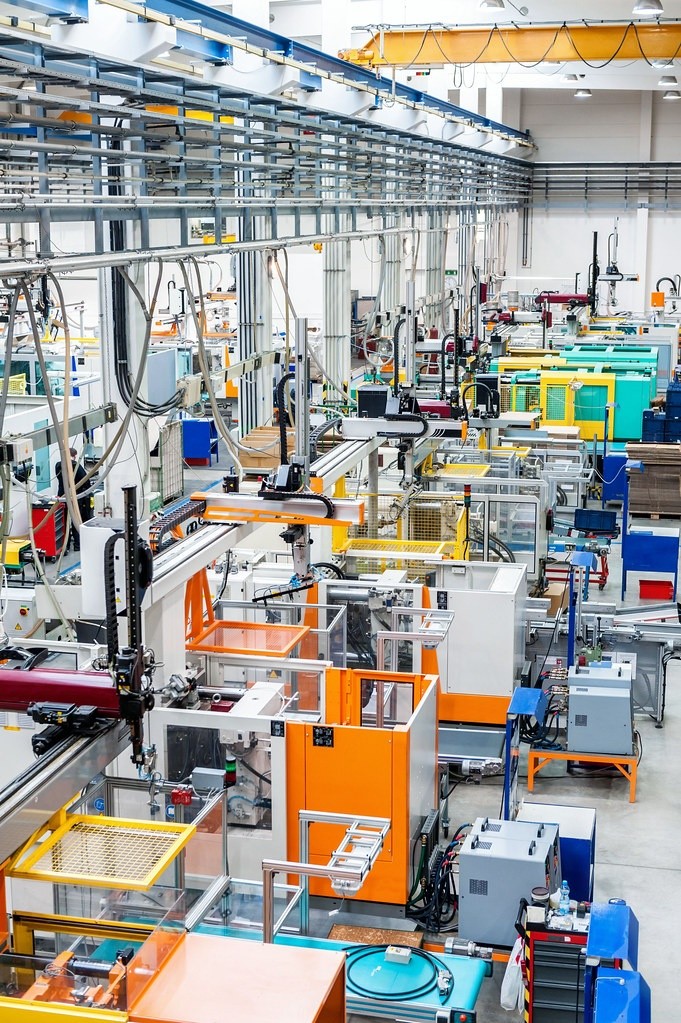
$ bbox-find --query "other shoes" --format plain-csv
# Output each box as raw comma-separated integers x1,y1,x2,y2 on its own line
74,548,80,551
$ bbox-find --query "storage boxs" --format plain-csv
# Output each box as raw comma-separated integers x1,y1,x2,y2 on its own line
529,582,570,615
238,426,295,468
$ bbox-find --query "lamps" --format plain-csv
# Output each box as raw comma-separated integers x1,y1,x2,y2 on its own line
658,76,678,85
663,91,681,99
560,75,579,83
479,0,505,12
574,89,592,97
651,59,675,68
632,0,664,15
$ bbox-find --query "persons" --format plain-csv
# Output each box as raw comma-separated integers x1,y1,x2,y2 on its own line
55,448,91,551
